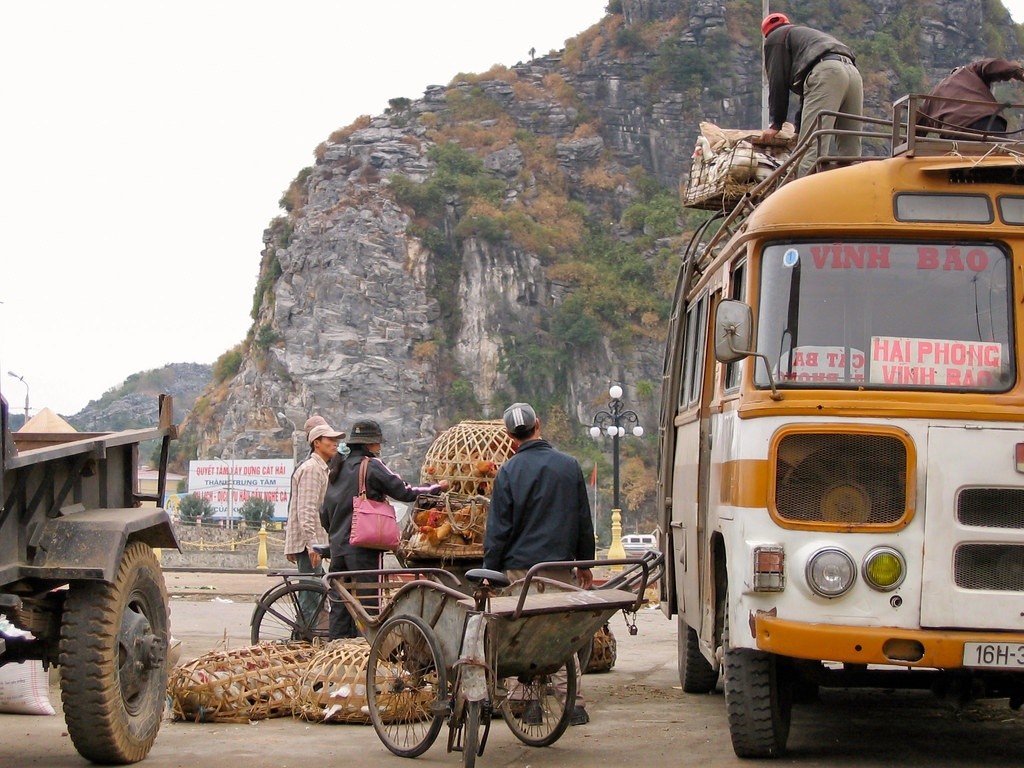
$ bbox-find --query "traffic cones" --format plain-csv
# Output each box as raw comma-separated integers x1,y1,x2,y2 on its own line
219,515,223,529
174,513,179,525
196,512,202,526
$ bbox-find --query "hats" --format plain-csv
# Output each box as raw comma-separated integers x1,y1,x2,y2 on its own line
761,13,790,37
344,419,388,444
503,403,537,434
308,425,346,446
304,416,330,436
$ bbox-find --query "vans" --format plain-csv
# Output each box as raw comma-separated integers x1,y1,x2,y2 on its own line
617,534,659,556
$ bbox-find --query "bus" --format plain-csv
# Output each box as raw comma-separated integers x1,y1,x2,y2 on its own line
649,104,1019,760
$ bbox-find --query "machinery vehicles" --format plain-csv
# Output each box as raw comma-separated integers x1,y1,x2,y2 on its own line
0,385,183,765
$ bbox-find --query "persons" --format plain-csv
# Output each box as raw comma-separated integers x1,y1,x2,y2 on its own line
287,414,346,641
319,419,448,640
904,57,1024,143
481,402,595,725
761,12,863,179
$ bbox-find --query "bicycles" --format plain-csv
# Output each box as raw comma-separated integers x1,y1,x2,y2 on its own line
248,539,356,652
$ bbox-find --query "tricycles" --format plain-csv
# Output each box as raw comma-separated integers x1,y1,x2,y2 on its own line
326,556,649,762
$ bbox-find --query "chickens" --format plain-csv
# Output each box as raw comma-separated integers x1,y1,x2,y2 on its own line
408,436,520,546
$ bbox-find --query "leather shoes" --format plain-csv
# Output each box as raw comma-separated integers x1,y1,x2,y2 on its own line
522,706,542,725
569,706,589,726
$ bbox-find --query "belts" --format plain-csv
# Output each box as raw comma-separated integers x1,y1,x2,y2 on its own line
816,55,853,65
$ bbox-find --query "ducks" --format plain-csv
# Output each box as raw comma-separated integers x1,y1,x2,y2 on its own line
182,639,434,721
685,133,798,189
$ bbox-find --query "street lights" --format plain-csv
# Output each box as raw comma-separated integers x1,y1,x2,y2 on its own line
591,384,643,582
8,372,30,426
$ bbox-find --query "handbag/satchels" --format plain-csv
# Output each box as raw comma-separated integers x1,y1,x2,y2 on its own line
348,459,401,551
